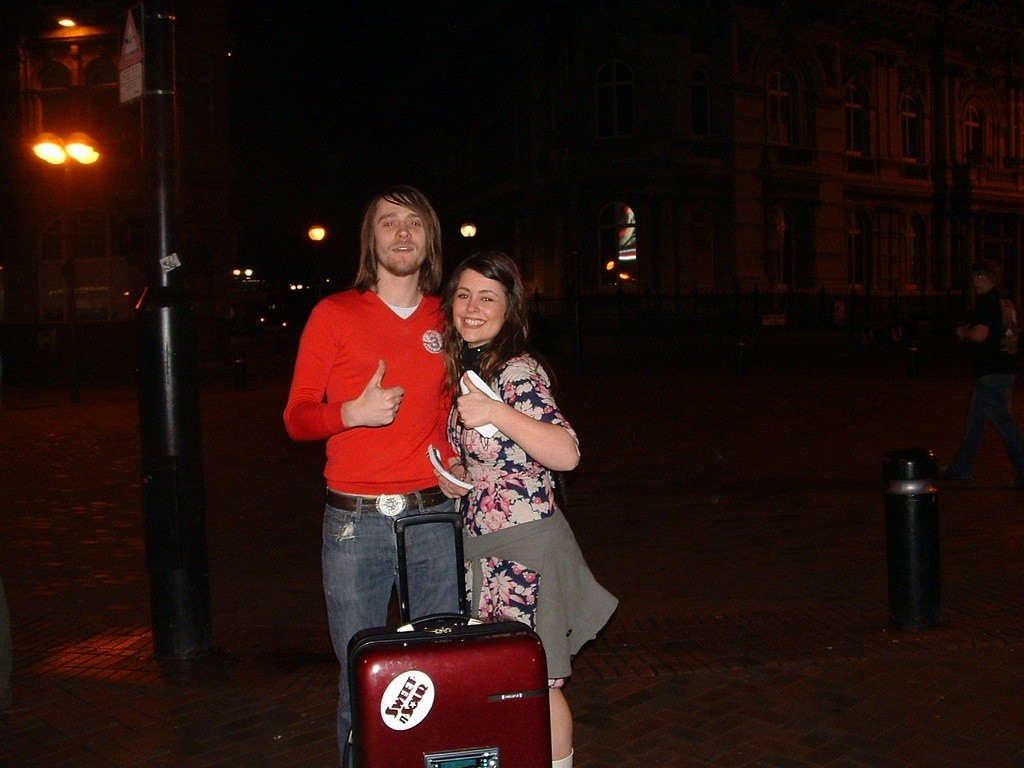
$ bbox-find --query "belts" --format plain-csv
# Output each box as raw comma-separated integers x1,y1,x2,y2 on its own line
324,486,449,518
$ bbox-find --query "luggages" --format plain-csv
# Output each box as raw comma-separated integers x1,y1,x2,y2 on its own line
343,511,553,768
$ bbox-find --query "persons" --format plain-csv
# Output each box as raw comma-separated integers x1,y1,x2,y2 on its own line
281,186,468,768
443,250,581,768
946,258,1024,489
